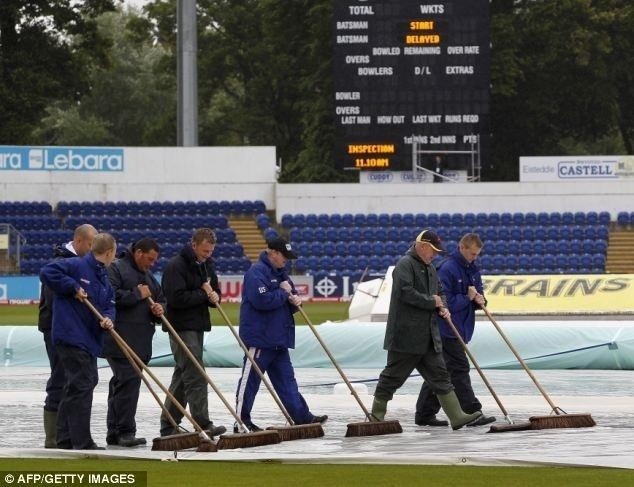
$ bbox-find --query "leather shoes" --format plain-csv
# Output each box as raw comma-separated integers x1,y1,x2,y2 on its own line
414,412,448,427
105,434,117,444
466,413,497,427
117,430,148,447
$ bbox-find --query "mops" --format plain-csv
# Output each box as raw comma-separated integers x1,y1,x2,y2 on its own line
76,289,215,451
474,294,597,428
206,291,325,440
436,299,542,431
138,284,281,449
288,291,403,437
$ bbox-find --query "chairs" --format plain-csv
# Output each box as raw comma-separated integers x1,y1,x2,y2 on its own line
0,196,634,276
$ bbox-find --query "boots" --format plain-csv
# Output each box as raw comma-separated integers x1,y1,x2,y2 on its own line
370,395,388,421
43,405,61,449
433,389,483,430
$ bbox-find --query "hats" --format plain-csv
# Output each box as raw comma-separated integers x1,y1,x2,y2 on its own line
267,237,297,260
413,228,443,251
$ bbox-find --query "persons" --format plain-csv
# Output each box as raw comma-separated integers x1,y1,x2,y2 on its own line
432,156,443,184
232,238,328,433
106,237,169,448
368,229,483,431
37,223,99,449
38,231,117,449
160,227,227,438
414,232,496,428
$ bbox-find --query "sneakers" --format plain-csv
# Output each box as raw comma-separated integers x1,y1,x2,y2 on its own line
233,419,265,434
206,421,227,436
310,412,329,427
87,441,105,449
